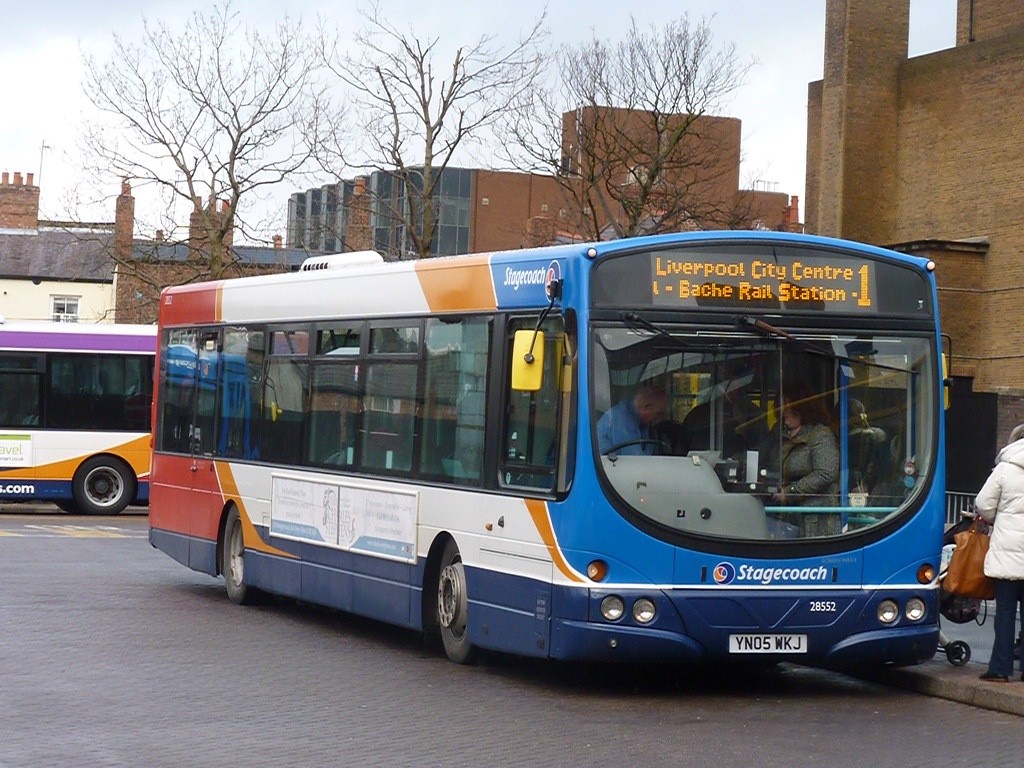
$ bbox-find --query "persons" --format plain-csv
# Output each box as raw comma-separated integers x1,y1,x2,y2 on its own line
768,381,839,537
974,425,1024,682
833,399,889,505
597,385,664,455
682,364,772,467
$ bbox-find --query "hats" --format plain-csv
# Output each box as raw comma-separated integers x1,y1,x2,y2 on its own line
835,397,866,419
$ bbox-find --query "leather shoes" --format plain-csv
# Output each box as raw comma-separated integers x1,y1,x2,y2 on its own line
979,667,1007,682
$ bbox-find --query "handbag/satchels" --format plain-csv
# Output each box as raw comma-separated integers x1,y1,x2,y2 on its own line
939,513,995,626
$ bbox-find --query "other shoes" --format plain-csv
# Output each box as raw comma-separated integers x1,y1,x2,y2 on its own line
1014,638,1022,659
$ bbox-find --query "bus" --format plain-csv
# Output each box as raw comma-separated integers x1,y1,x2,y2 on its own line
0,320,159,517
168,342,263,462
147,228,954,668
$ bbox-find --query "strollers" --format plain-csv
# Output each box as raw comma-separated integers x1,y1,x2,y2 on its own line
936,513,993,666
